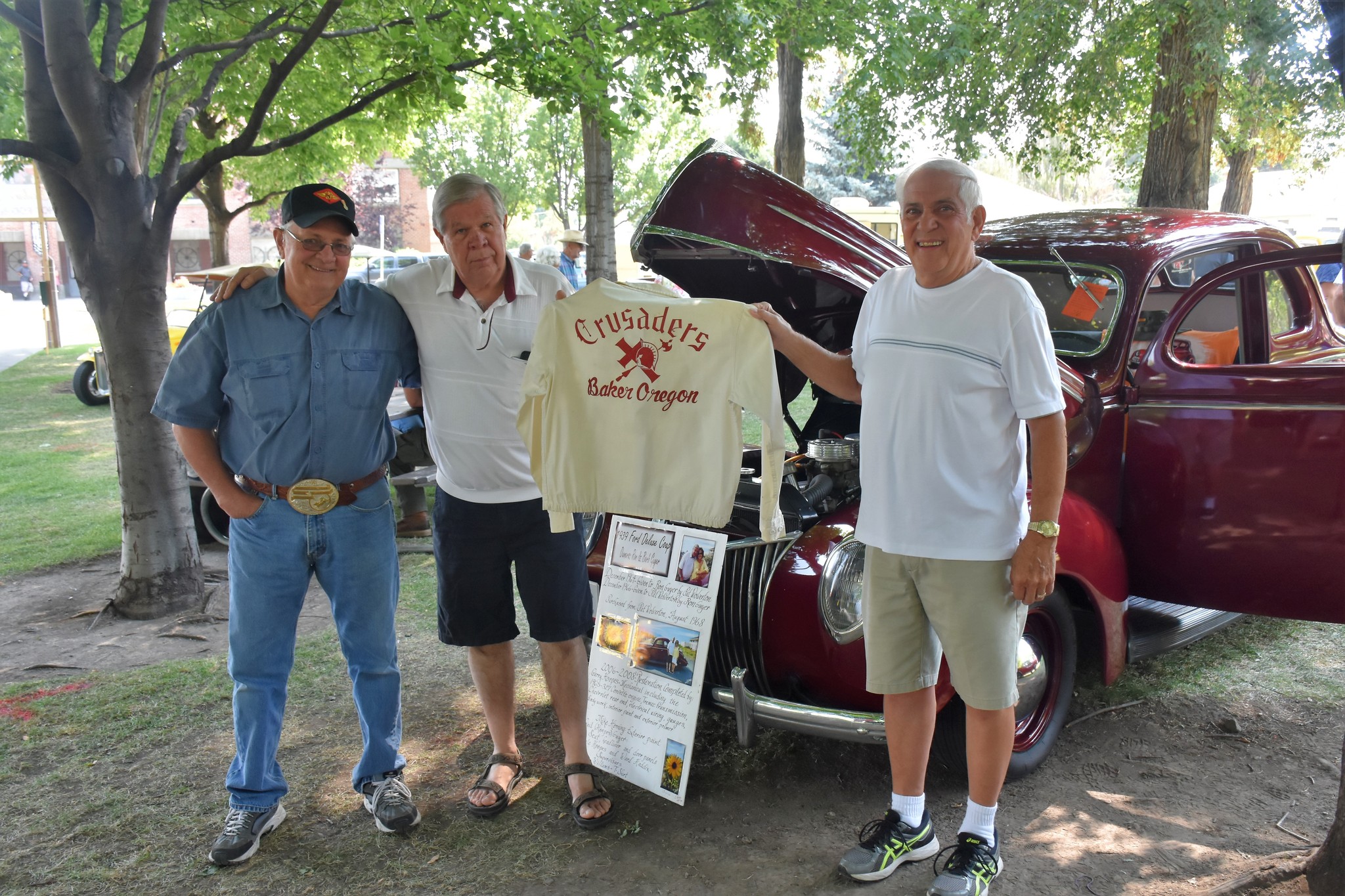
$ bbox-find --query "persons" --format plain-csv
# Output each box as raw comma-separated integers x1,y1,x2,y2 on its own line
750,156,1068,895
666,637,680,674
678,544,709,582
152,184,424,868
518,230,590,293
208,175,618,832
17,261,34,301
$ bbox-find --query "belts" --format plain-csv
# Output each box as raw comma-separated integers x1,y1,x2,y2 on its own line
236,464,389,514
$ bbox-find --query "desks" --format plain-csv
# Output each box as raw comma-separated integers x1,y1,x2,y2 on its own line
386,387,420,434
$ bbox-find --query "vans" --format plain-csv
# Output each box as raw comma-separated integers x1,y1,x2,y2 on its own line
343,253,449,287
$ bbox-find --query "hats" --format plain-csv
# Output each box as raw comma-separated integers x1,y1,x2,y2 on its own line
557,229,588,246
281,184,358,236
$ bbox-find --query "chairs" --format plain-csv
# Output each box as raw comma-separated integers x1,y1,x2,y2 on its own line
1133,309,1238,372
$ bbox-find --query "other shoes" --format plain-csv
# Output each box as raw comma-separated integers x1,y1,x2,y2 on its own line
392,515,433,538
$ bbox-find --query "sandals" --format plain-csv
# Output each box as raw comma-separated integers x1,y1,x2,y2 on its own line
562,758,618,829
468,746,525,814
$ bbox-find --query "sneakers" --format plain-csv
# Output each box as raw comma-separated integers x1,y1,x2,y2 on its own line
837,810,939,880
927,828,1003,896
362,772,421,832
210,803,287,864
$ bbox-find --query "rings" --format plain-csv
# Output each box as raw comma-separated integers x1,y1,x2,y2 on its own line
1036,592,1046,598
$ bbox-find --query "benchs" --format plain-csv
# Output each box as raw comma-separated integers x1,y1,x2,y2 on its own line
388,463,437,553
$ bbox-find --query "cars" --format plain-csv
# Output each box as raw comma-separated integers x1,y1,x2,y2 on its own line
631,133,1345,793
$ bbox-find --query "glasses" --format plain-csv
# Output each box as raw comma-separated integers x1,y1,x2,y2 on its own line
277,228,356,255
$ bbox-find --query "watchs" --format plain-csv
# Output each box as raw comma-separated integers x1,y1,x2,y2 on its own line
1027,520,1060,538
